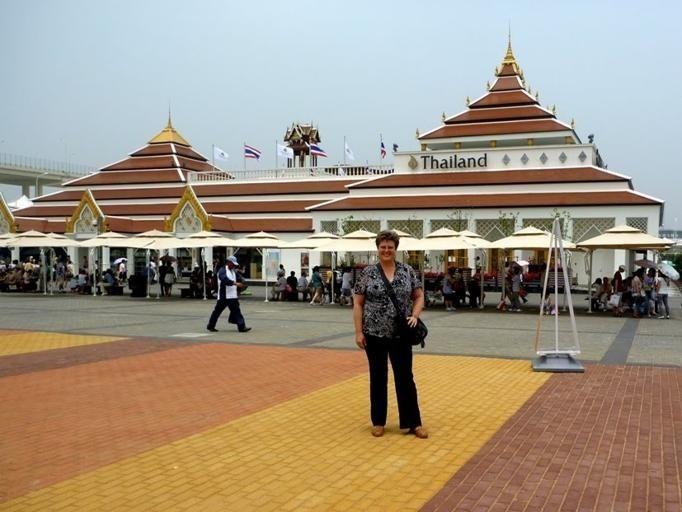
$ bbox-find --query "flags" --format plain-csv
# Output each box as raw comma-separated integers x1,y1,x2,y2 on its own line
344,142,354,160
212,146,229,161
310,143,327,158
278,145,294,160
381,141,387,161
245,146,261,160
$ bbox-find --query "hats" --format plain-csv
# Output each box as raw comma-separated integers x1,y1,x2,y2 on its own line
226,255,240,267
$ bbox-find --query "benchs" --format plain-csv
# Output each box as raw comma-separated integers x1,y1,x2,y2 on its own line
75,281,127,296
1,280,40,293
177,286,200,298
280,289,332,303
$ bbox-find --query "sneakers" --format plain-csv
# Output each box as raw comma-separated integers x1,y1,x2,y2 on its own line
309,301,354,306
445,306,457,312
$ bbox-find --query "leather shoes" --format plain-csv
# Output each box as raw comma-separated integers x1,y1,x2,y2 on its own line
508,306,524,313
206,326,220,333
372,426,387,438
411,425,429,439
585,306,671,320
238,326,252,334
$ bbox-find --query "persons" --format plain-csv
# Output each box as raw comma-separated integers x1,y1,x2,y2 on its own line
585,265,670,319
352,230,428,437
1,257,252,332
411,257,567,313
269,263,366,306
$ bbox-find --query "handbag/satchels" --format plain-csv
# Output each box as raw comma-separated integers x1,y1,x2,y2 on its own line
394,313,428,348
609,293,621,309
